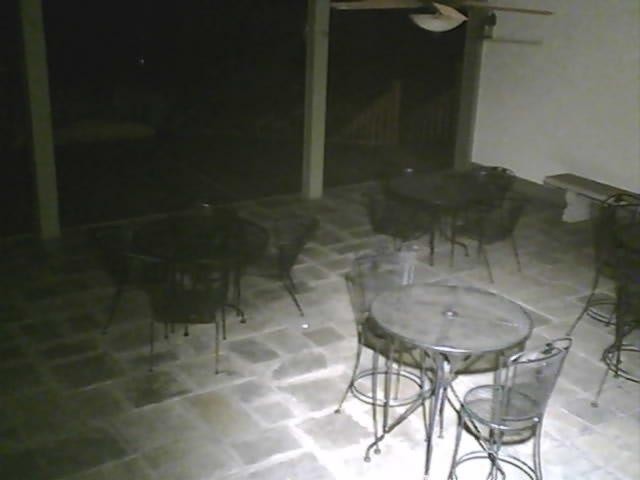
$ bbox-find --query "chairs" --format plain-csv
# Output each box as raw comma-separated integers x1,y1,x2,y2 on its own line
446,334,570,479
93,205,320,374
566,194,640,408
334,247,435,456
369,161,531,283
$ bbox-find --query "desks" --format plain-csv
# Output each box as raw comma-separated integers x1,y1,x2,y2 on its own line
363,283,535,478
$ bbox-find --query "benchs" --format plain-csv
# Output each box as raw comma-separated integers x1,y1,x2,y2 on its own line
545,173,640,208
